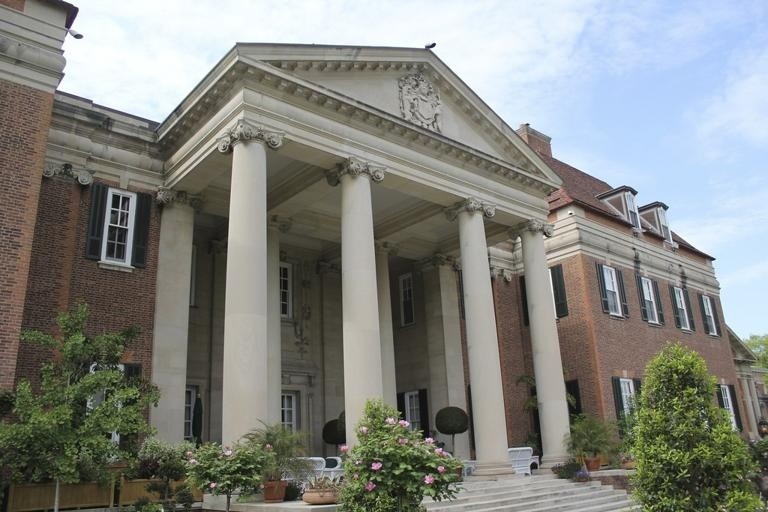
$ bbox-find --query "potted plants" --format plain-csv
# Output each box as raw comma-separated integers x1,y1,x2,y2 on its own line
238,418,340,503
562,395,619,471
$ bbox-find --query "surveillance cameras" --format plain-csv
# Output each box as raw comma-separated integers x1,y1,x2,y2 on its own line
425,42,436,48
568,211,573,215
69,29,84,39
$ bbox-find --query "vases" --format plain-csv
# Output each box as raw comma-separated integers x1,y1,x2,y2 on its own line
577,478,588,482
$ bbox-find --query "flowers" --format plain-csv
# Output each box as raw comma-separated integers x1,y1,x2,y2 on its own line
575,471,590,477
